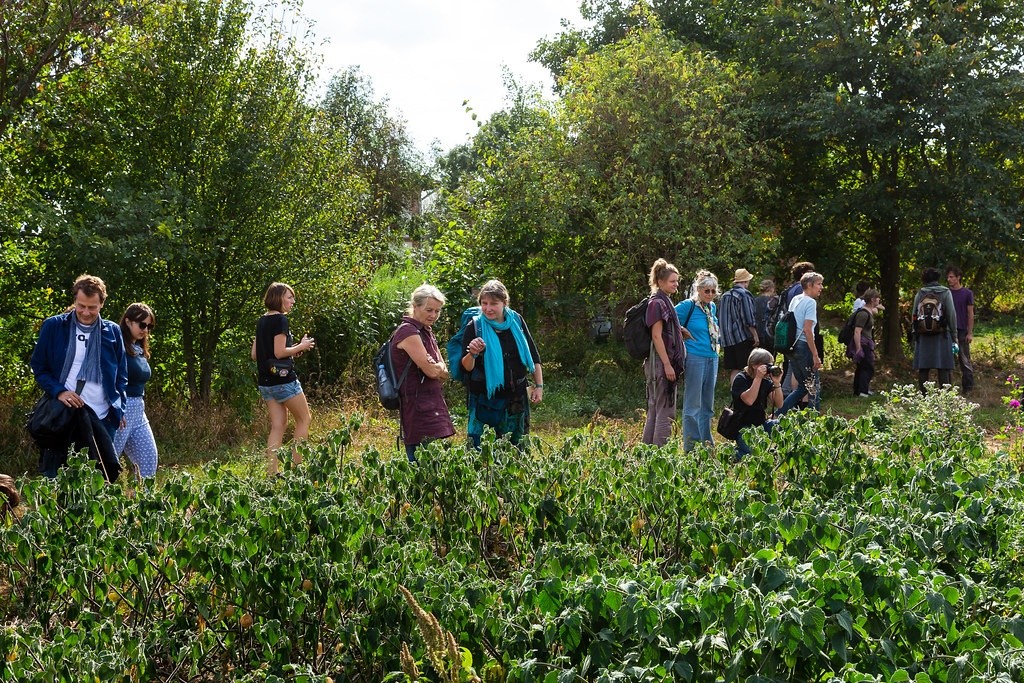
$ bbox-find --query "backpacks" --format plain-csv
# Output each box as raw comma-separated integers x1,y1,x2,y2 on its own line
773,297,804,353
838,308,870,345
376,322,422,409
913,289,950,334
623,295,672,360
766,282,800,337
447,306,522,382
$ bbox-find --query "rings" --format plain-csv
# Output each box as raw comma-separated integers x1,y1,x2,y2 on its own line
68,398,72,401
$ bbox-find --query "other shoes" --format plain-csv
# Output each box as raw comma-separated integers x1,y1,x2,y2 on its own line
866,390,874,395
853,393,868,398
794,402,809,413
729,454,739,463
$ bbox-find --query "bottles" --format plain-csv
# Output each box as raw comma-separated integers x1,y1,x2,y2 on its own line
778,310,785,320
951,340,960,354
913,311,916,320
379,365,393,398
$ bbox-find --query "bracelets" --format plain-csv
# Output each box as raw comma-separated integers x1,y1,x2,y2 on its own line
681,326,683,329
773,384,781,388
535,384,544,388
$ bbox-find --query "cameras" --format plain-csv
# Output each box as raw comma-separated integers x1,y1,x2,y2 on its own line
767,362,782,376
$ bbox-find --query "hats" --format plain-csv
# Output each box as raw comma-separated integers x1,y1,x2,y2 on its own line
733,269,753,282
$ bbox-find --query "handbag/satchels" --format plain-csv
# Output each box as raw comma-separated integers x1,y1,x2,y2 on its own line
475,393,507,427
258,317,292,377
26,393,79,449
717,408,744,440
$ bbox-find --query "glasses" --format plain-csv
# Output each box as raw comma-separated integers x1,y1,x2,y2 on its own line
702,289,716,294
139,322,154,330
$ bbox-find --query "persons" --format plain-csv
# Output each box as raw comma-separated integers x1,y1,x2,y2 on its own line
458,280,544,454
729,348,780,465
31,274,129,486
638,259,696,450
845,280,885,398
768,272,824,422
753,279,780,357
944,266,974,399
672,269,721,456
773,262,815,399
912,269,959,399
387,283,456,463
112,302,158,498
251,282,315,483
718,268,759,385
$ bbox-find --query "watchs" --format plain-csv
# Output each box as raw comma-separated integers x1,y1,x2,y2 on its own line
469,351,480,359
